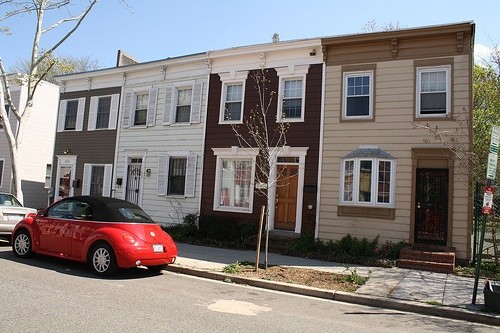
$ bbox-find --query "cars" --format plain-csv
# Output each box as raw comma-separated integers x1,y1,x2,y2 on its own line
9,195,178,278
0,192,37,246
